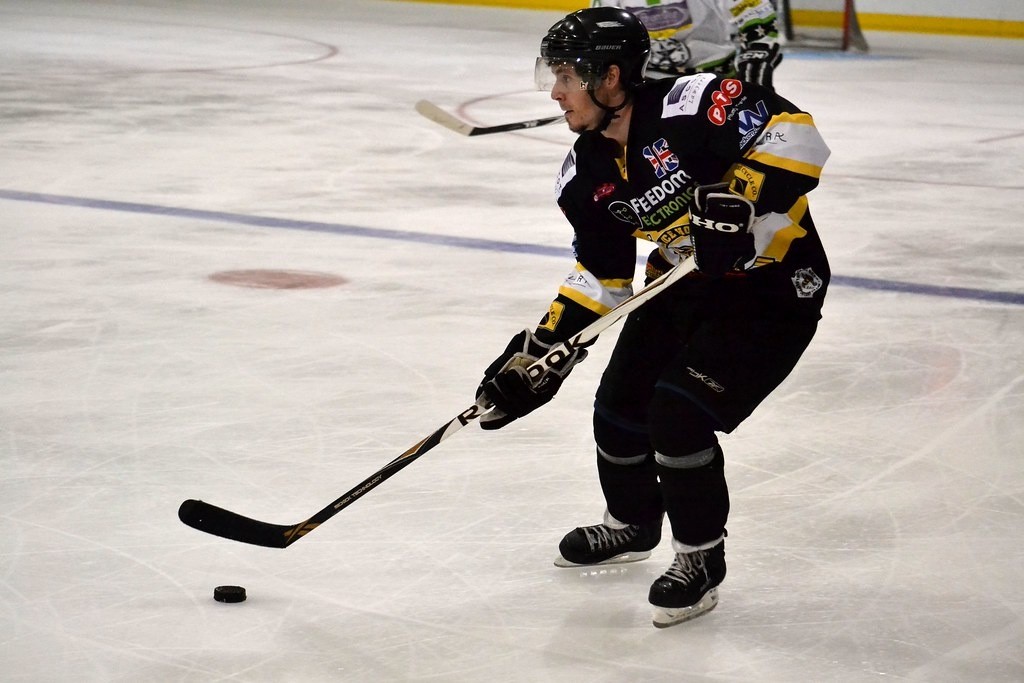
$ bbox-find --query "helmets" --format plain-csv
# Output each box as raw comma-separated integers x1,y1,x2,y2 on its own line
541,7,651,88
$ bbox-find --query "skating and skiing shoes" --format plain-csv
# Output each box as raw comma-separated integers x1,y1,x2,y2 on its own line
649,533,726,628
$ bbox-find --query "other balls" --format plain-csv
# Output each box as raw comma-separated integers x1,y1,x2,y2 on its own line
213,584,247,606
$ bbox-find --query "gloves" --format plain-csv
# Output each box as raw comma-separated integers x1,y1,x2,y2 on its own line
688,181,756,278
736,41,782,86
476,287,614,429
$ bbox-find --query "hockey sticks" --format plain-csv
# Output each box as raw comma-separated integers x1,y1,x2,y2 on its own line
176,247,696,552
412,61,737,139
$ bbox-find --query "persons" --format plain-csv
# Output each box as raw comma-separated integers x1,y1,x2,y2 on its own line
475,7,833,629
590,0,786,93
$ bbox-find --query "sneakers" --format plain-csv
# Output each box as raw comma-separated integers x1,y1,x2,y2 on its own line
555,509,663,567
